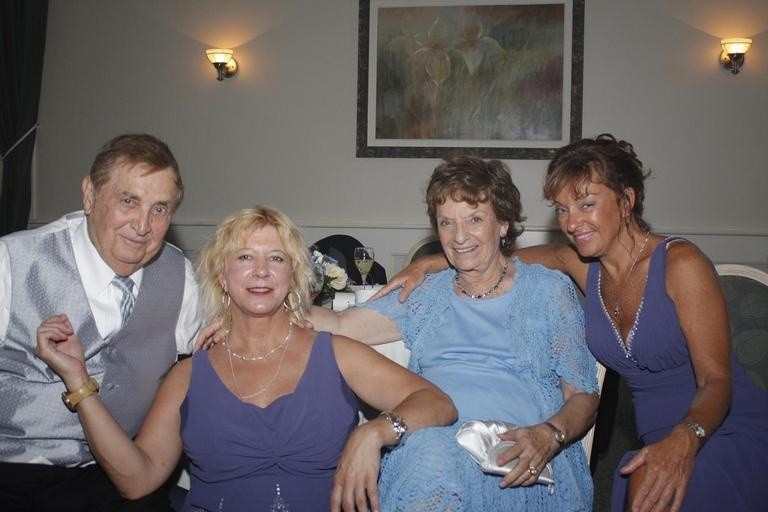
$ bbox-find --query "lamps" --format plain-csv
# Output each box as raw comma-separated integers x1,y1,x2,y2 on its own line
720,37,752,74
206,48,238,81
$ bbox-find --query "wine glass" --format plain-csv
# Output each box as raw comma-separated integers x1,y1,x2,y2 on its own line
354,247,375,289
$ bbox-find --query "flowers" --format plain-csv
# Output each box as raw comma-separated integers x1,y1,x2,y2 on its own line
310,250,348,304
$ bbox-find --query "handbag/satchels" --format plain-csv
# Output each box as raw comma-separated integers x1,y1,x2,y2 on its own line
455,421,555,497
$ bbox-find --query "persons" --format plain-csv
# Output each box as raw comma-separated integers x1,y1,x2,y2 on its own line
305,154,602,509
367,134,766,512
33,206,458,510
0,133,313,511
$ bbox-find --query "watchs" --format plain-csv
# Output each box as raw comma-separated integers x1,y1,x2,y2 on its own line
679,417,706,447
544,420,564,451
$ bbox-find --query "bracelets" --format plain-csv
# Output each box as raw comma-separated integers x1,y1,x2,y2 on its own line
60,378,99,412
378,408,406,450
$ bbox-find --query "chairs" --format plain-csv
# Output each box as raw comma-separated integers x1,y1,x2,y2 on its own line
713,263,768,390
404,235,444,272
579,361,606,466
308,234,383,282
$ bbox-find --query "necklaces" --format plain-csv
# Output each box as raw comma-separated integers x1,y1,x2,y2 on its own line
456,262,509,299
606,230,649,319
219,322,292,361
225,322,292,399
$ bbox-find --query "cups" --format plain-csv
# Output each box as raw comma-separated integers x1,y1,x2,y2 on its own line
355,290,375,303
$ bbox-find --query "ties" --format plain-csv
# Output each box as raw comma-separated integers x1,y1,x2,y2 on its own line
112,274,136,327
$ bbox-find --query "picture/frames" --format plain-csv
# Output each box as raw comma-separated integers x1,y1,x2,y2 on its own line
357,0,584,159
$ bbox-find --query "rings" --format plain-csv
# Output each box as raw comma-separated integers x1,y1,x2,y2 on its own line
528,466,538,476
